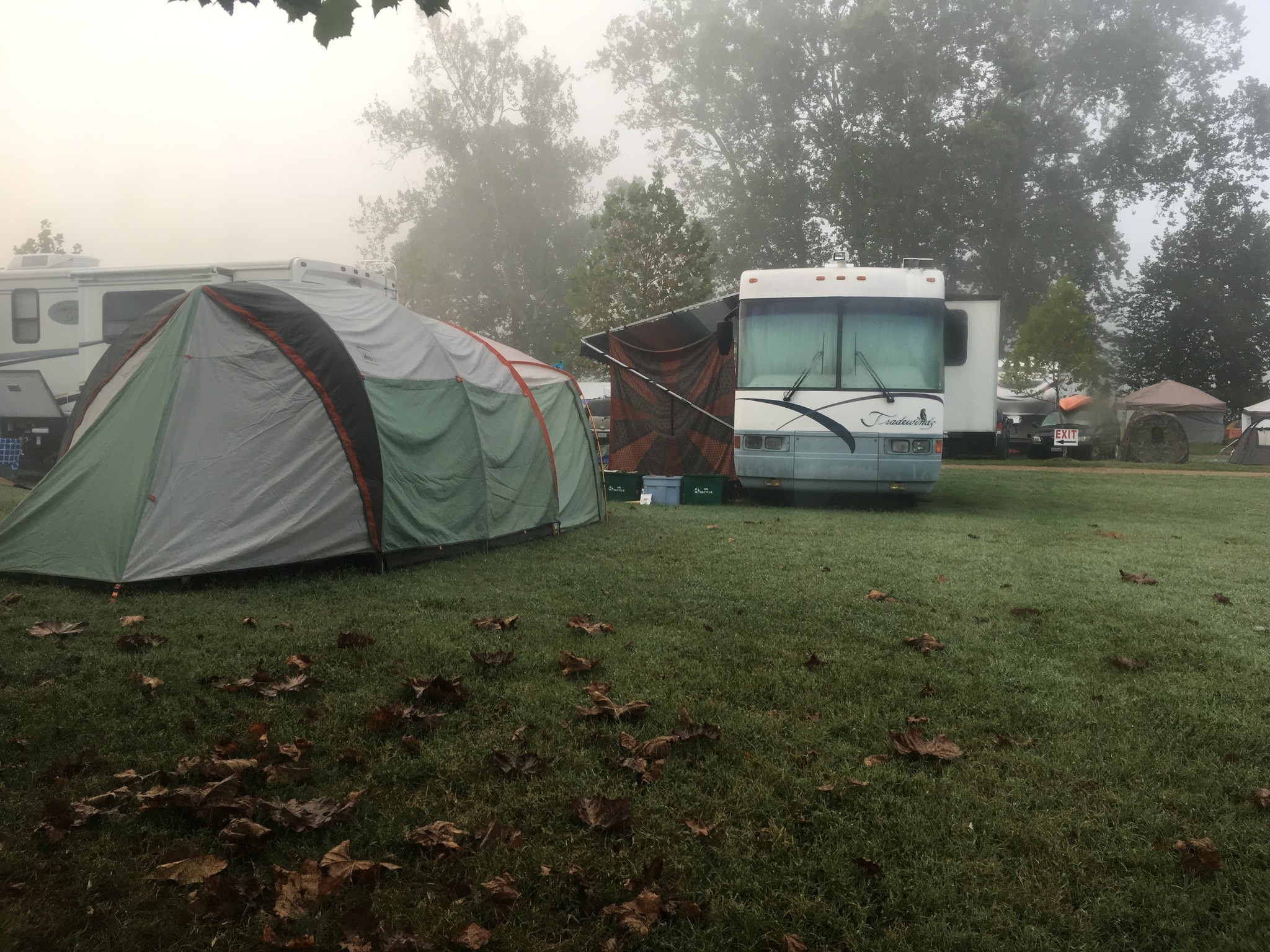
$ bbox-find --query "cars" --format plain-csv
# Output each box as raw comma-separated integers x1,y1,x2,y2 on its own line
994,407,1014,460
583,395,611,447
1029,398,1122,461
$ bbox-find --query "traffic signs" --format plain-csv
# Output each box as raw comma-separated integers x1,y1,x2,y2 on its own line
1054,429,1078,446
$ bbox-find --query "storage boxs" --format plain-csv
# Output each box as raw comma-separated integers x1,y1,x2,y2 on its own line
599,471,645,502
682,473,730,506
642,475,684,506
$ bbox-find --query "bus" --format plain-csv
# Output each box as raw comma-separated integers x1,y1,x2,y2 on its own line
1,251,399,449
729,256,944,500
